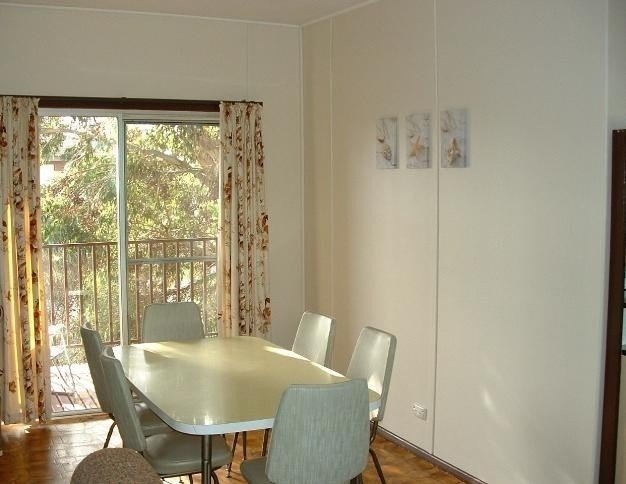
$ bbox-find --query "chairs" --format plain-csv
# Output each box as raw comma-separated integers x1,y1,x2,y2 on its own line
346,326,398,484
80,322,193,484
240,378,370,484
227,311,336,478
101,345,233,484
143,301,205,344
